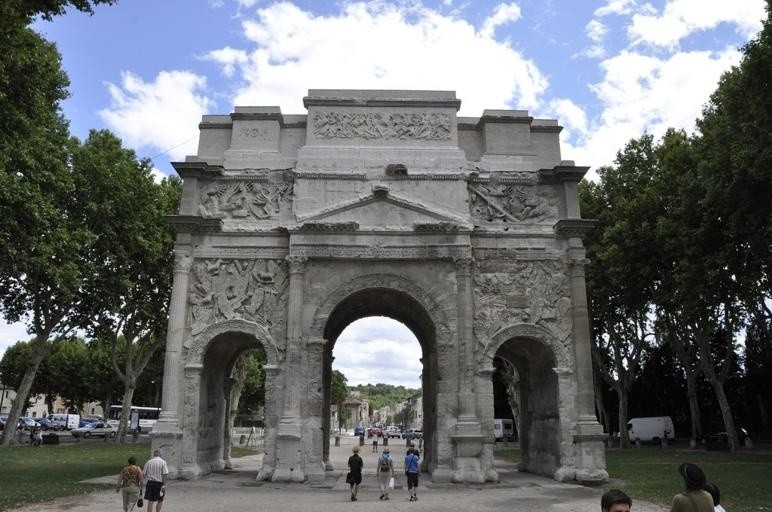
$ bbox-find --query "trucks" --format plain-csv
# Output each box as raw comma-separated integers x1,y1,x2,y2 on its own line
616,416,677,446
493,418,515,441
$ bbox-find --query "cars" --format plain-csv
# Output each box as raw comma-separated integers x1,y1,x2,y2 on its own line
0,412,118,448
354,422,424,439
702,425,750,452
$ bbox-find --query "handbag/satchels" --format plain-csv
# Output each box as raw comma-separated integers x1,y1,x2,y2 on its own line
137,489,143,507
346,472,352,483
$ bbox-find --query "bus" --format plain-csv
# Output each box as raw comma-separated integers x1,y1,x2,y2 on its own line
105,403,164,437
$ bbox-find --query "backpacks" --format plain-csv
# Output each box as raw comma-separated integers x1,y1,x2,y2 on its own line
381,454,390,472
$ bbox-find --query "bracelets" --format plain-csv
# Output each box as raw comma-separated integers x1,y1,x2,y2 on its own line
377,471,379,471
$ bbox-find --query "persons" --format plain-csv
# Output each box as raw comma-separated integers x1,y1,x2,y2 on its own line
348,446,362,500
376,446,395,501
30,422,41,445
117,455,144,511
370,431,379,453
670,462,715,511
406,443,419,456
34,429,42,445
404,447,420,500
382,427,389,445
142,449,168,511
702,481,725,511
601,489,631,511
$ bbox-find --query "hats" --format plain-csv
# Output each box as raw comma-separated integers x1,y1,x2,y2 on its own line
679,463,706,490
